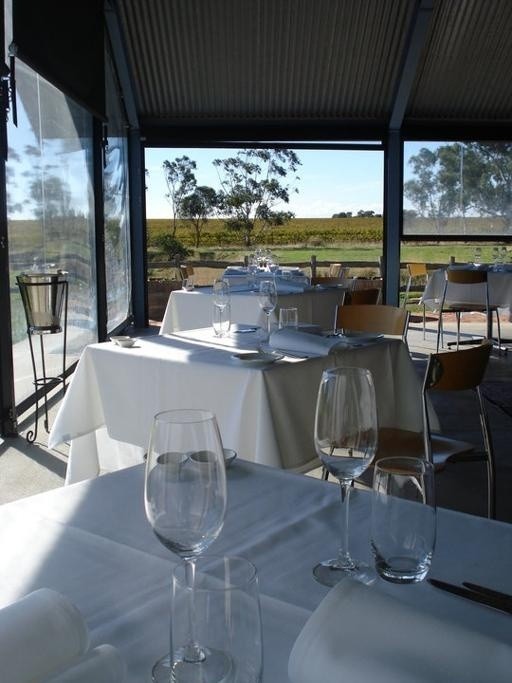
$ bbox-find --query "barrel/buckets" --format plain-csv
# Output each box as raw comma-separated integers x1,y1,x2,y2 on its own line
19,271,70,334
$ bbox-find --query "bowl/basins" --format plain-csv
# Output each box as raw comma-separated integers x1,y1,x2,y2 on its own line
191,447,237,473
110,333,135,347
156,451,189,473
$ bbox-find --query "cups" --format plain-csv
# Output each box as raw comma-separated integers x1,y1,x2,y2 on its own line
169,555,265,683
211,303,231,335
449,255,455,265
182,278,194,291
279,307,299,331
367,456,437,585
329,263,345,287
309,366,378,589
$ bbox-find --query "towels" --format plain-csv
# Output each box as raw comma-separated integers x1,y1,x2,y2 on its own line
285,567,512,683
0,588,127,683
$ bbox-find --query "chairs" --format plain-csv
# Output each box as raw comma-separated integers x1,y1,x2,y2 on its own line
178,255,501,356
322,345,496,519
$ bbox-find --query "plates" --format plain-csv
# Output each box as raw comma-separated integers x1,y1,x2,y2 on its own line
232,351,284,364
286,323,321,330
339,332,385,342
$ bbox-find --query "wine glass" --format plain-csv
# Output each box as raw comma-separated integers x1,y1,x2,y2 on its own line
244,250,298,292
147,407,227,683
472,247,507,272
257,281,279,352
213,279,229,339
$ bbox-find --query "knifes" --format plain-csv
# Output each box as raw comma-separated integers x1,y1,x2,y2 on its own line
427,575,511,618
